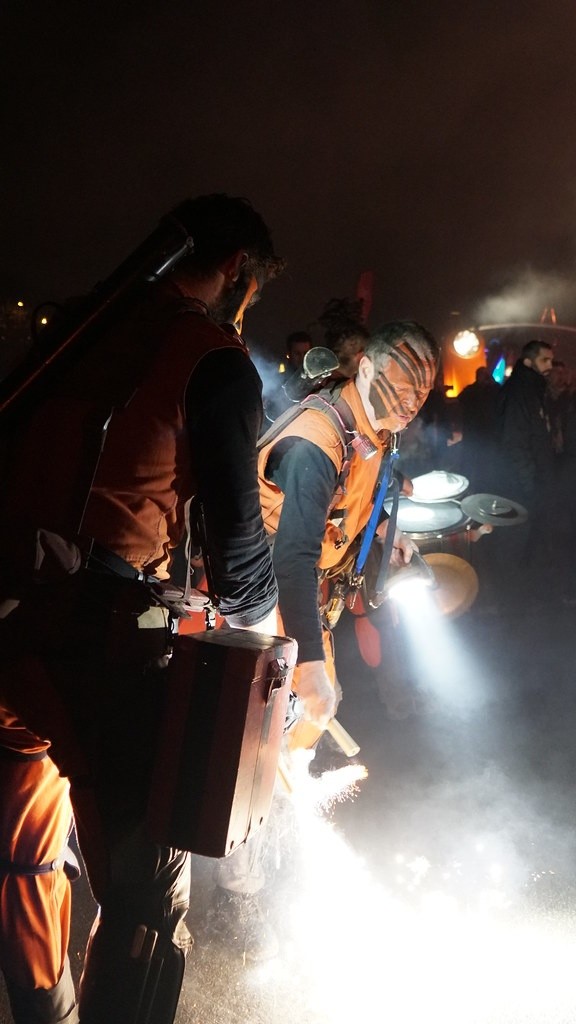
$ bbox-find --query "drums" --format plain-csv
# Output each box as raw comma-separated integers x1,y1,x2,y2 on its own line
382,495,473,567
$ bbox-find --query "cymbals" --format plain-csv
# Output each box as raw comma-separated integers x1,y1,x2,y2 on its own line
407,470,530,526
422,551,480,622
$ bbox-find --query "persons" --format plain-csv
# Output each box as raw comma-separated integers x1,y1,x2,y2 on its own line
0,190,576,1024
